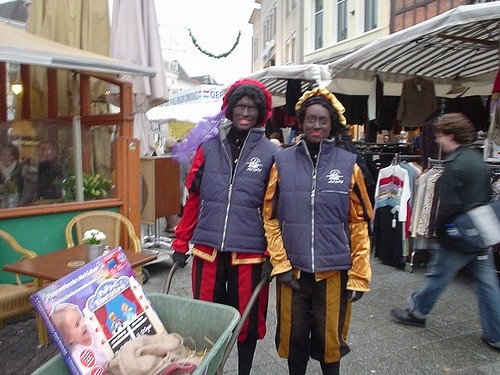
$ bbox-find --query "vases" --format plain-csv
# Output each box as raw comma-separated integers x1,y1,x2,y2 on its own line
7,192,18,207
85,243,102,261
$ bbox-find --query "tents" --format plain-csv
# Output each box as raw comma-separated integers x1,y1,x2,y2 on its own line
147,0,500,159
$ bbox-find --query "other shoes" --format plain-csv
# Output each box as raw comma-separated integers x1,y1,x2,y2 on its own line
391,308,426,327
480,334,500,352
164,223,180,237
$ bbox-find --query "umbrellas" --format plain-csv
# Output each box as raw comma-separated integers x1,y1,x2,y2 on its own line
11,0,170,244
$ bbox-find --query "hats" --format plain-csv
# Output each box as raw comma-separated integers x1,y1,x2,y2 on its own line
295,87,347,127
270,139,283,148
221,79,272,127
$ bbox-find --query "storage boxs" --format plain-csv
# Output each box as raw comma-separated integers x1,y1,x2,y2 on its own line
29,246,168,375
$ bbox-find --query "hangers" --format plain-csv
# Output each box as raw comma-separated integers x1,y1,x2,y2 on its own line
408,72,433,81
352,143,425,167
427,158,447,175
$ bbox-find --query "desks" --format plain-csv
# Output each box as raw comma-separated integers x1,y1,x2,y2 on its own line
3,249,156,346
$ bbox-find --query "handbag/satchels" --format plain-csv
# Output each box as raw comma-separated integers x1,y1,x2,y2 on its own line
454,200,500,253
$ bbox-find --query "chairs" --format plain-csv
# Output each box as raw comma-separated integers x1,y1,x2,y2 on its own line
65,212,142,285
0,231,49,349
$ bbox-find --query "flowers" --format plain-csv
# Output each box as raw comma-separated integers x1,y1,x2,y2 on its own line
6,181,18,194
83,229,105,247
54,174,112,203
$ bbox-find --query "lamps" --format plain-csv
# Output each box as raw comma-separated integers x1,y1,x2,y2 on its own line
11,82,22,96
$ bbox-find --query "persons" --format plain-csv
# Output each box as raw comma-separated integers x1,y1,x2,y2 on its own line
262,86,373,375
51,303,107,375
391,113,500,353
171,78,280,375
